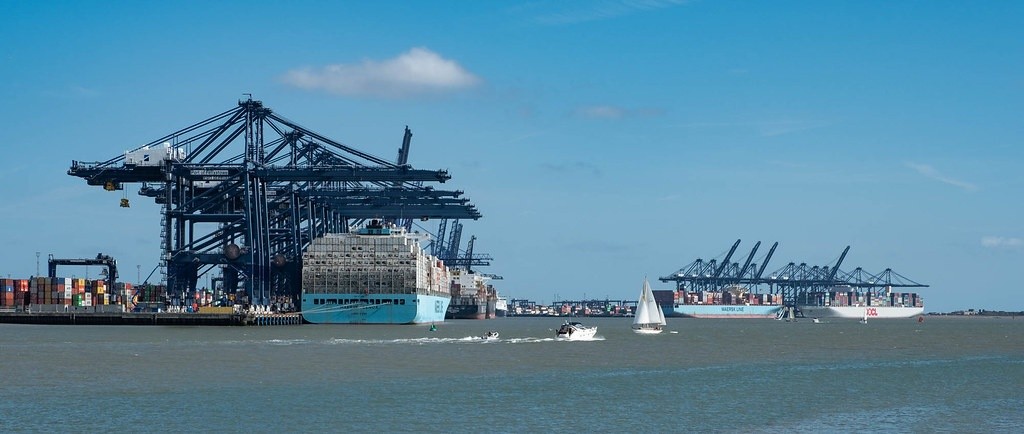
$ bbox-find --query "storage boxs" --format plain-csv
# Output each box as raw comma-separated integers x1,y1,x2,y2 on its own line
655,290,923,308
0,277,289,310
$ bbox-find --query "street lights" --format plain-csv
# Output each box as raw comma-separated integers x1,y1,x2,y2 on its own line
36,251,40,277
137,265,140,287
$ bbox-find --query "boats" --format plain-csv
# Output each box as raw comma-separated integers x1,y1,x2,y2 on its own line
661,303,786,319
796,303,924,318
555,319,598,341
446,267,508,320
481,332,499,339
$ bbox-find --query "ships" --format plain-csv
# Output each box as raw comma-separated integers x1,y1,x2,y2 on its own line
301,219,452,324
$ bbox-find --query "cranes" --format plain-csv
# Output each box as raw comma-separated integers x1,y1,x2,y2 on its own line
658,239,929,310
67,93,503,312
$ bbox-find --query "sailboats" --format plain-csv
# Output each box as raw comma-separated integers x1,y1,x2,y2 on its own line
631,275,667,334
